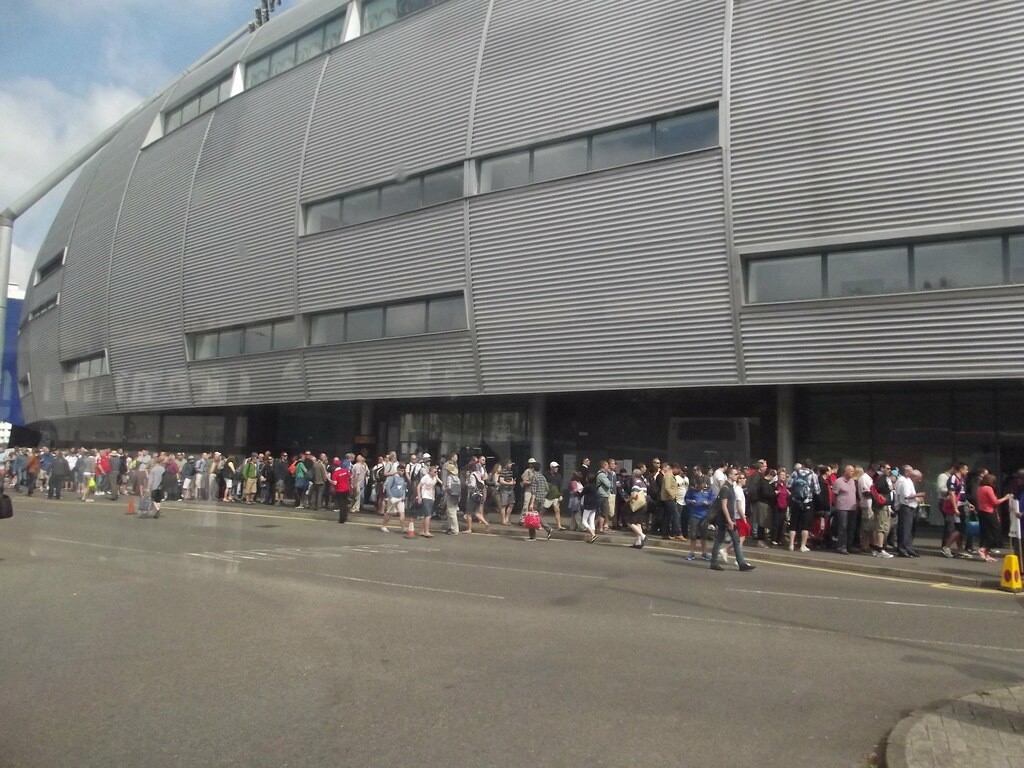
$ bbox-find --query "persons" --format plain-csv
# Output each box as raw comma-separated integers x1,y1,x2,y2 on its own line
0,446,567,541
569,456,1024,571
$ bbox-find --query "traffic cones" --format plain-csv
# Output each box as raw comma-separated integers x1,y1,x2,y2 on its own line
124,495,138,515
998,554,1021,594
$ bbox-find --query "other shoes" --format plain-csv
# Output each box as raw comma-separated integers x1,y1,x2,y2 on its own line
183,495,361,513
378,520,757,572
15,483,135,502
756,538,1004,562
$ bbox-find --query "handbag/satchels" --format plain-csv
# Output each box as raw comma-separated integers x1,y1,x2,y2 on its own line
295,478,307,488
520,507,540,528
138,471,178,511
566,486,647,518
88,477,96,491
790,470,981,543
706,484,729,522
305,468,314,481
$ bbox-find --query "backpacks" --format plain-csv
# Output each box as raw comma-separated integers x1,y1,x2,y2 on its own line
371,462,485,504
129,460,138,470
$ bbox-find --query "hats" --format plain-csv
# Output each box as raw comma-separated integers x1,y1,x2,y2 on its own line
109,451,118,457
528,458,537,463
252,452,258,456
550,461,560,467
